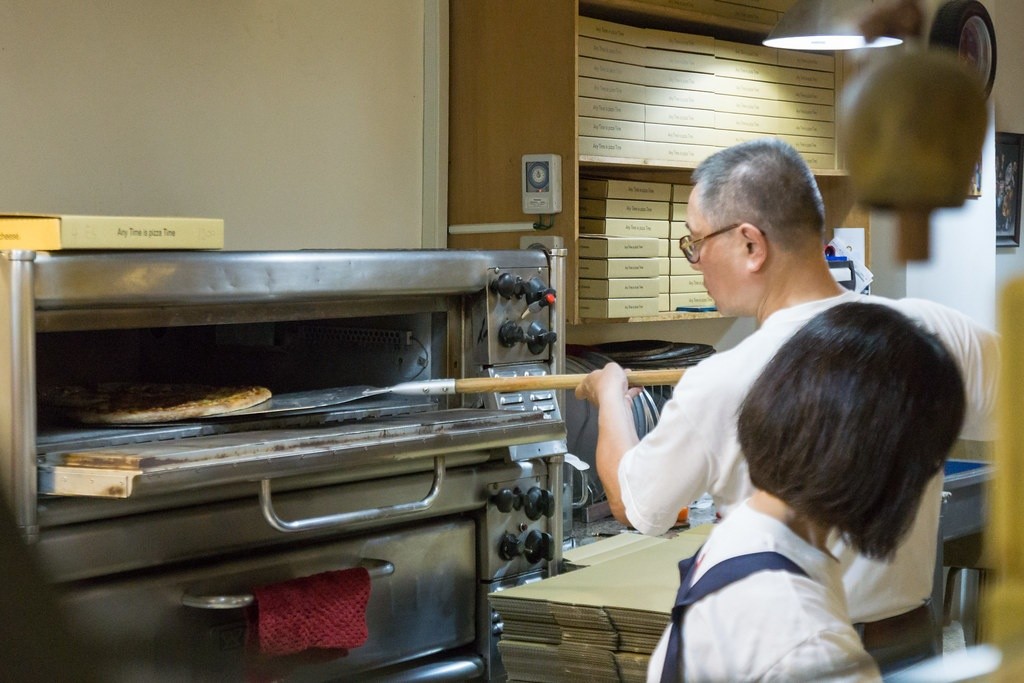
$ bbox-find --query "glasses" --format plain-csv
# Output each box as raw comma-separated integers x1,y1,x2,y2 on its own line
680,225,765,265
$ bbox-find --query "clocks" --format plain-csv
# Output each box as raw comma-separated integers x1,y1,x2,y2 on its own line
928,0,998,105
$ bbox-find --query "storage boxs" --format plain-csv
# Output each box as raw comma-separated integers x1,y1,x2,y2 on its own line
576,13,838,320
0,211,225,251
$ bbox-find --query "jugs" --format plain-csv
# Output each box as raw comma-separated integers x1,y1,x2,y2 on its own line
559,452,591,543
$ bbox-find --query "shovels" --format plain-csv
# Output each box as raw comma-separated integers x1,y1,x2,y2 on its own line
200,365,684,419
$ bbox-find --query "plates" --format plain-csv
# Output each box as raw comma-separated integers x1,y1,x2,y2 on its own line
564,343,668,507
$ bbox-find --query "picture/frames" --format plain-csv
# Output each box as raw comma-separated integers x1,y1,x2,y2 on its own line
995,131,1024,247
964,146,982,197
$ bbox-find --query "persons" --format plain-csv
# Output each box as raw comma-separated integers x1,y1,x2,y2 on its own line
646,300,967,682
576,137,1002,681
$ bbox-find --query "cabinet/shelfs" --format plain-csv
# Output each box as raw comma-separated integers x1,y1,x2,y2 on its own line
448,0,871,329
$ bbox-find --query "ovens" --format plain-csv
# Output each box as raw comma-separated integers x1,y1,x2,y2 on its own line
0,247,569,683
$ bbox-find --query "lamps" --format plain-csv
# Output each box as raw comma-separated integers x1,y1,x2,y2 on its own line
762,1,904,51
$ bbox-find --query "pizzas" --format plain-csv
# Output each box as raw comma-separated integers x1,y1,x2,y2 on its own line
36,380,273,424
